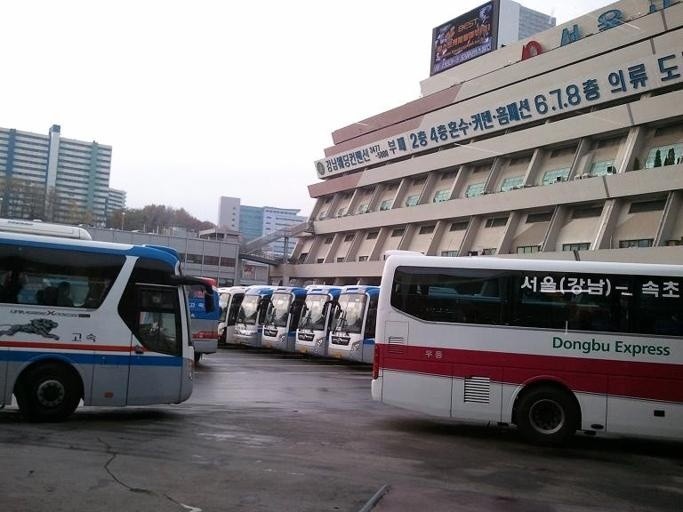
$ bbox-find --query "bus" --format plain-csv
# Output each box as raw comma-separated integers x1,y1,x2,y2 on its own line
218,284,381,365
182,275,223,360
370,249,682,446
1,219,195,422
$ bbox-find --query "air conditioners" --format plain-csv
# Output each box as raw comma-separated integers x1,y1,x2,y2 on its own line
551,166,616,183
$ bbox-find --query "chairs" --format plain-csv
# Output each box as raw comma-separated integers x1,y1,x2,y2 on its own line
35,289,47,305
43,287,57,305
609,292,627,332
504,275,528,326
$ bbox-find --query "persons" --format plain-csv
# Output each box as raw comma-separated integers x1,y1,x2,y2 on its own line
55,282,76,307
431,5,491,64
